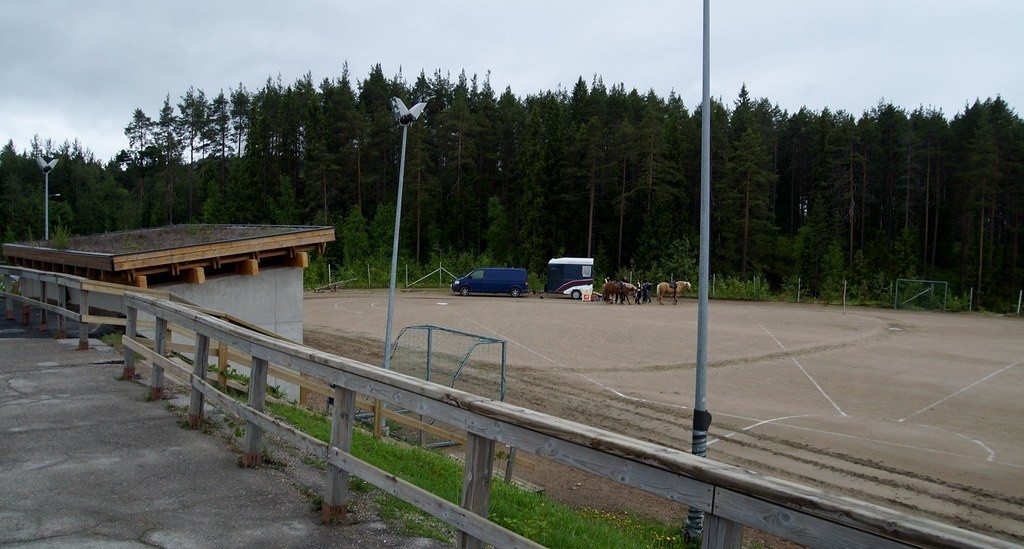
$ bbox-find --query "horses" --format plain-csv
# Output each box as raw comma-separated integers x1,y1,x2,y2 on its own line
656,281,691,305
604,282,623,304
622,282,640,305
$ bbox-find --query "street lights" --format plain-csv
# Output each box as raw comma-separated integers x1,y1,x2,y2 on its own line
36,156,59,240
382,97,428,371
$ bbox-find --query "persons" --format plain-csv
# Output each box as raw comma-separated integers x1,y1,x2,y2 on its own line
635,279,652,305
605,276,628,304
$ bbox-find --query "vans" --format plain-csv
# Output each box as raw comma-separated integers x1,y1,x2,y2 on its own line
451,268,530,297
